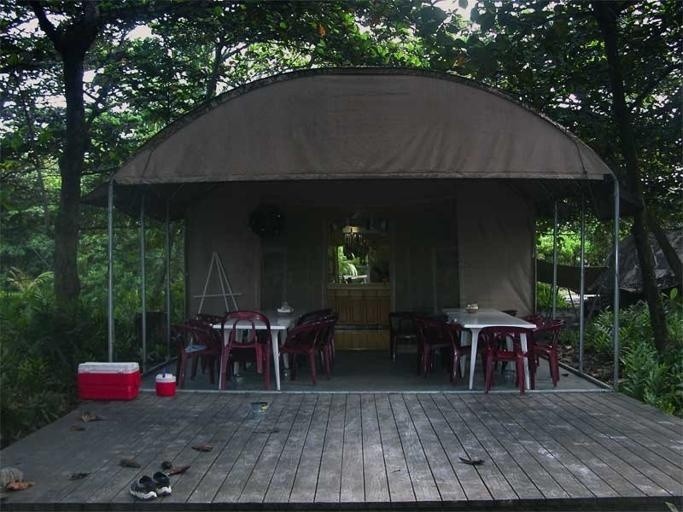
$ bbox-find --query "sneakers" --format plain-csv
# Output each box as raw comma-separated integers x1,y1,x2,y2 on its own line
129,472,172,500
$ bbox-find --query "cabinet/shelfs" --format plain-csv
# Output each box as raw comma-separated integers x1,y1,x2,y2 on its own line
350,290,378,324
327,289,352,324
377,290,391,324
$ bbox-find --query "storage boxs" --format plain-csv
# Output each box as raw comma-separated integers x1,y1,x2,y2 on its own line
155,367,176,397
76,361,141,401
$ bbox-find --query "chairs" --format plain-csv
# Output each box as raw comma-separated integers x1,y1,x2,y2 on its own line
389,309,566,395
170,304,340,391
